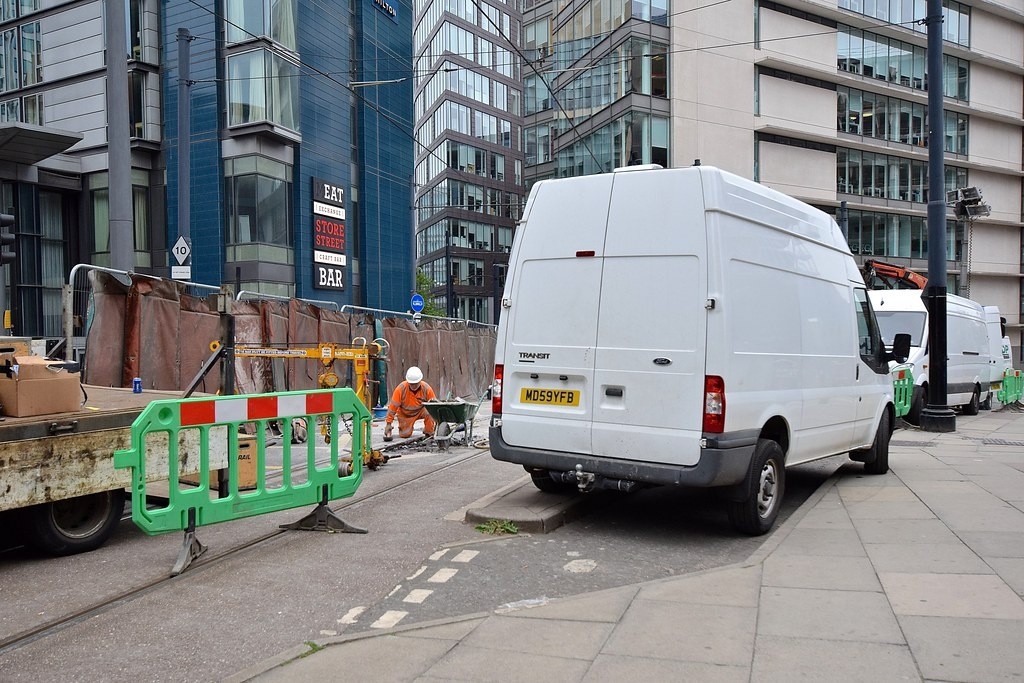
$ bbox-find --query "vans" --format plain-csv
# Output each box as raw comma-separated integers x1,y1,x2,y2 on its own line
1002,336,1013,377
854,289,1004,416
487,160,912,536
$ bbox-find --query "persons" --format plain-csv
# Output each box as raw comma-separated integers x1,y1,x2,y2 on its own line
384,366,438,438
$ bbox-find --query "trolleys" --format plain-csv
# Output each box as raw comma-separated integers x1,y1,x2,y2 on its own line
422,384,493,451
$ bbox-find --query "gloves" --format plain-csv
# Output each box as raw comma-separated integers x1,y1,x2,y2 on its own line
384,422,392,435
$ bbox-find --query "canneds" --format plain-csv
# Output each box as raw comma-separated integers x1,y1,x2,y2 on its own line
133,378,142,393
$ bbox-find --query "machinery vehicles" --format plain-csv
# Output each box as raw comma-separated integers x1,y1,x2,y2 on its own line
859,259,928,290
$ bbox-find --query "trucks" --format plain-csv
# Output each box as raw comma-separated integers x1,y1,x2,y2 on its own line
0,382,221,554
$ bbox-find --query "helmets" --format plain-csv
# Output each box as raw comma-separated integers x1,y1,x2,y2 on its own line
405,366,424,383
